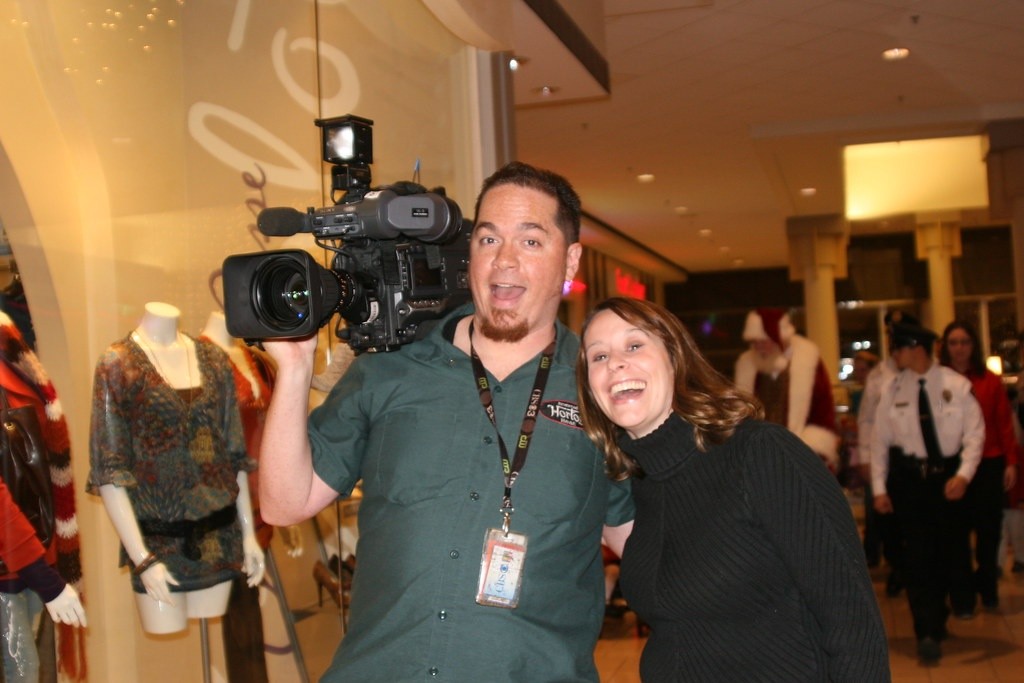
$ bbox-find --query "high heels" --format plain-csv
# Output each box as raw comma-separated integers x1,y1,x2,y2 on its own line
313,560,350,609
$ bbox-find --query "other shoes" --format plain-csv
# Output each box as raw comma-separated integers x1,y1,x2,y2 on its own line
953,596,973,615
918,635,941,663
981,584,997,609
887,570,903,597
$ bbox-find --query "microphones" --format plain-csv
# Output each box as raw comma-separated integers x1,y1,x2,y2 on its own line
258,207,314,236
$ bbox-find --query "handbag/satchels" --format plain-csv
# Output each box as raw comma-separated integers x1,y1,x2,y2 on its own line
0,388,55,549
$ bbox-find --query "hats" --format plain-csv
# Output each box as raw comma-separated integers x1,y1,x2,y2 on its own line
883,310,922,332
886,319,937,354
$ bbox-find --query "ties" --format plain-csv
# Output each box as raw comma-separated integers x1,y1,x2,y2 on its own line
918,379,945,474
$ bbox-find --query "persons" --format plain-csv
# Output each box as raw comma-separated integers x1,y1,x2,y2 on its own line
578,296,891,683
258,164,637,683
733,308,1024,666
0,301,280,683
